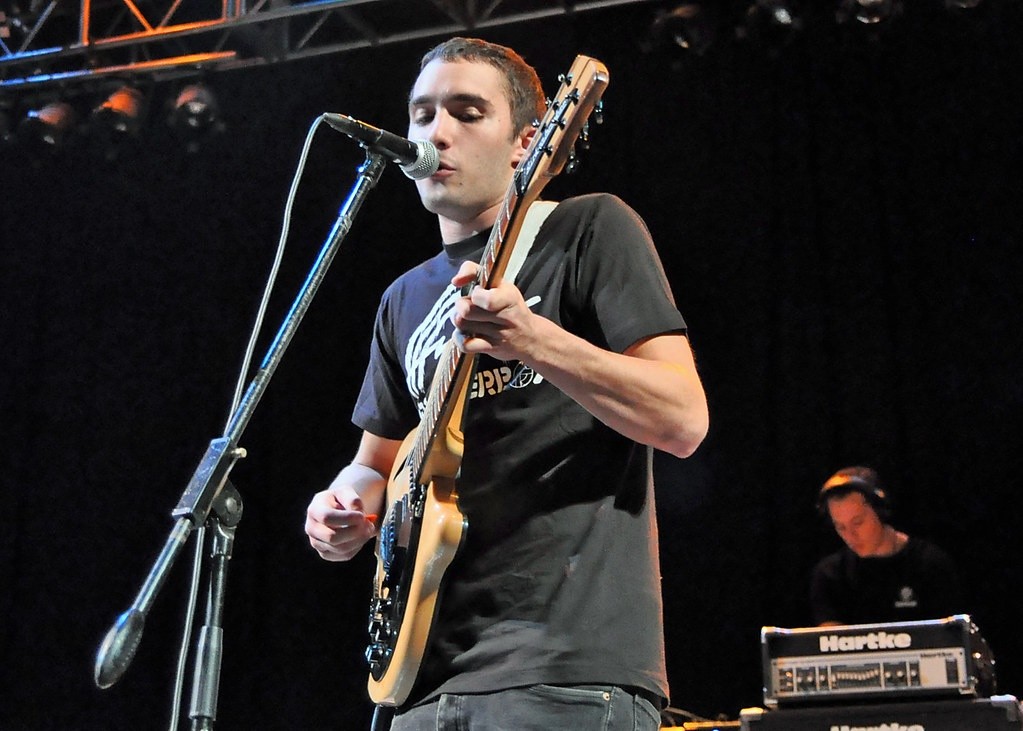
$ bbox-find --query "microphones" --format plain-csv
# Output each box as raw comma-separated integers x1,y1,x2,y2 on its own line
324,113,440,180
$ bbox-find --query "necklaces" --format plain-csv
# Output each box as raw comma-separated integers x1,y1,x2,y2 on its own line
891,529,897,554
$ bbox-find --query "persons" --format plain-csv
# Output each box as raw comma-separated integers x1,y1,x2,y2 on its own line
304,37,709,731
810,463,970,649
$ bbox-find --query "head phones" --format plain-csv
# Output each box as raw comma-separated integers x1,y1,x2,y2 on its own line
817,477,886,522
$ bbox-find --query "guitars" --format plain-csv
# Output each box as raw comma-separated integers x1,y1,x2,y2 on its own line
364,52,610,707
